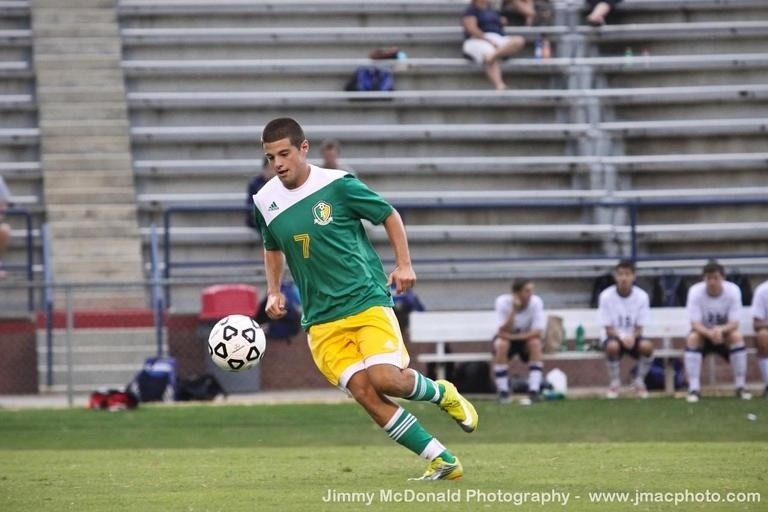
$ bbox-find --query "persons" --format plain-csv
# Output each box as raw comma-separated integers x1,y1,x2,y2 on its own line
1,173,14,280
751,280,768,399
683,264,753,403
581,0,625,25
459,1,526,90
245,154,287,266
317,140,359,177
501,0,538,51
597,259,654,400
489,277,548,405
253,118,479,482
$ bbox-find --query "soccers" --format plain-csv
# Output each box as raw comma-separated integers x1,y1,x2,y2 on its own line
208,314,266,371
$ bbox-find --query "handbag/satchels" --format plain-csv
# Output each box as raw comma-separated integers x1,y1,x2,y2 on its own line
253,284,303,341
89,357,224,411
343,69,396,92
645,357,690,391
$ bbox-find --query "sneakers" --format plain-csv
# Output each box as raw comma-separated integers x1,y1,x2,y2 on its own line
629,380,650,400
436,379,478,433
406,454,462,482
500,385,514,405
527,389,543,404
736,387,752,401
607,378,621,399
686,390,703,403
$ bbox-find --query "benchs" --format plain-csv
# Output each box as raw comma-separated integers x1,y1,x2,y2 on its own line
0,0,768,397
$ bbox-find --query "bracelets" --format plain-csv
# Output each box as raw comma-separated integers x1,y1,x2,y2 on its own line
719,324,726,335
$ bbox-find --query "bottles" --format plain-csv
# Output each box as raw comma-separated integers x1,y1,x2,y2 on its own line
533,33,551,59
576,324,584,352
